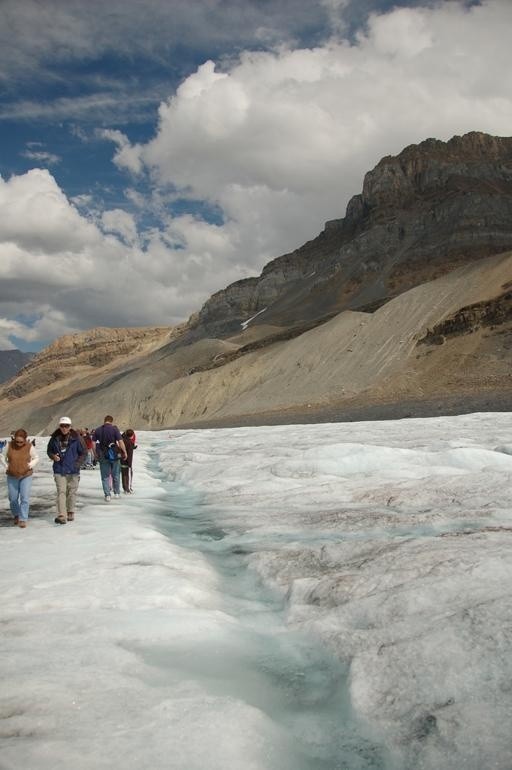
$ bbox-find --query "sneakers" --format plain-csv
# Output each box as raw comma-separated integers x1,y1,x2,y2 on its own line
105,487,134,502
14,515,27,528
67,512,74,521
55,516,66,524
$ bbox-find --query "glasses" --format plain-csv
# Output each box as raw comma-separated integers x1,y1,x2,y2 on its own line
60,424,69,428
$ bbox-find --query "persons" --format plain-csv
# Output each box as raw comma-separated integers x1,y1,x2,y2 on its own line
0,415,137,527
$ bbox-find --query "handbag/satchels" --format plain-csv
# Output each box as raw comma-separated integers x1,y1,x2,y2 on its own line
104,442,122,460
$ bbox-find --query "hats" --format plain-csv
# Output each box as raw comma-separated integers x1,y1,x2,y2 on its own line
59,417,71,425
